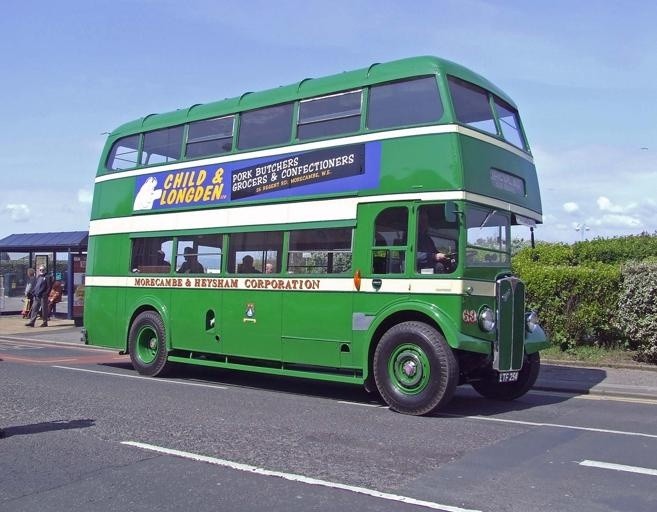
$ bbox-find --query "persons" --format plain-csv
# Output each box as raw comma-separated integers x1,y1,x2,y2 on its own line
177,246,203,274
20,267,35,319
263,262,273,273
23,264,49,327
239,255,261,274
398,208,455,274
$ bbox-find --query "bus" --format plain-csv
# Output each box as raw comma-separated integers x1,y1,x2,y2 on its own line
81,56,547,415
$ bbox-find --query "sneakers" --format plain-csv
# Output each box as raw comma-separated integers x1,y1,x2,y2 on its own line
25,322,34,326
36,317,50,321
39,324,47,327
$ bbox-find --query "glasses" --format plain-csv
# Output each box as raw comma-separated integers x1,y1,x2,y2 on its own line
40,269,44,271
